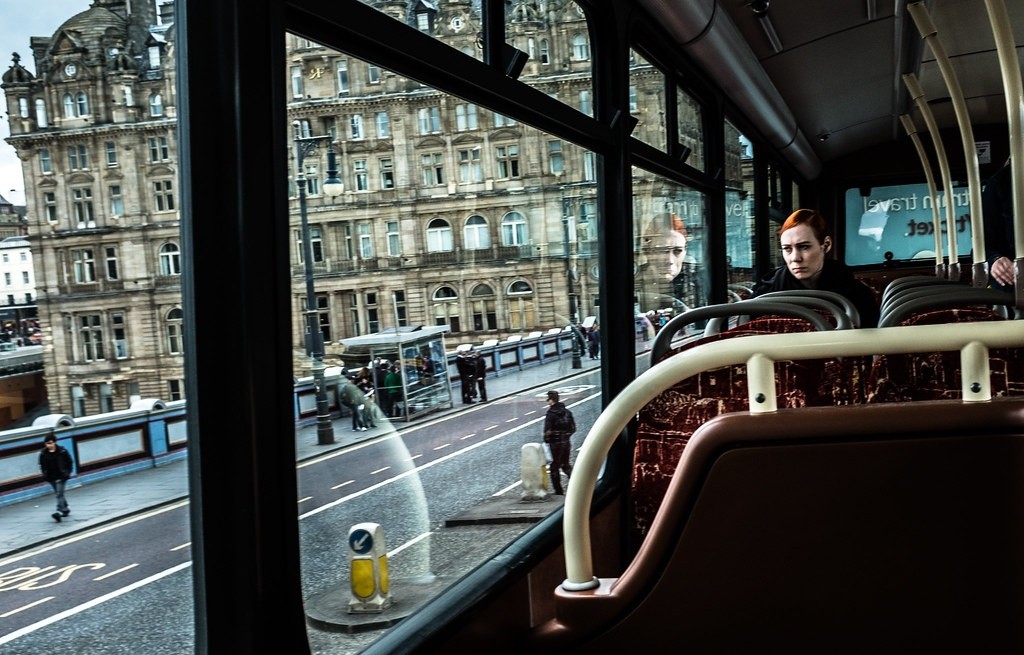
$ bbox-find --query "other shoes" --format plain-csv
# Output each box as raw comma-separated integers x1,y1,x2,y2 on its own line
352,430,356,431
51,513,61,522
365,425,369,428
60,509,71,517
361,427,368,430
479,400,487,402
472,402,475,403
371,425,376,427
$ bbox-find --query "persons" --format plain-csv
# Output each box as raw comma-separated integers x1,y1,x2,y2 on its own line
543,391,578,495
455,349,488,405
38,433,74,522
736,210,868,326
982,157,1017,286
634,214,703,314
332,345,444,432
572,301,707,360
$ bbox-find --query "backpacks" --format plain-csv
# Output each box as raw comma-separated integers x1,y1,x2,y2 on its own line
340,385,351,406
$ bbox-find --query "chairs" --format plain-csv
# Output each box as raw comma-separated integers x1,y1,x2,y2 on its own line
526,276,1024,655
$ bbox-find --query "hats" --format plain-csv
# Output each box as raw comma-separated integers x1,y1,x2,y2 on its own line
345,373,357,380
